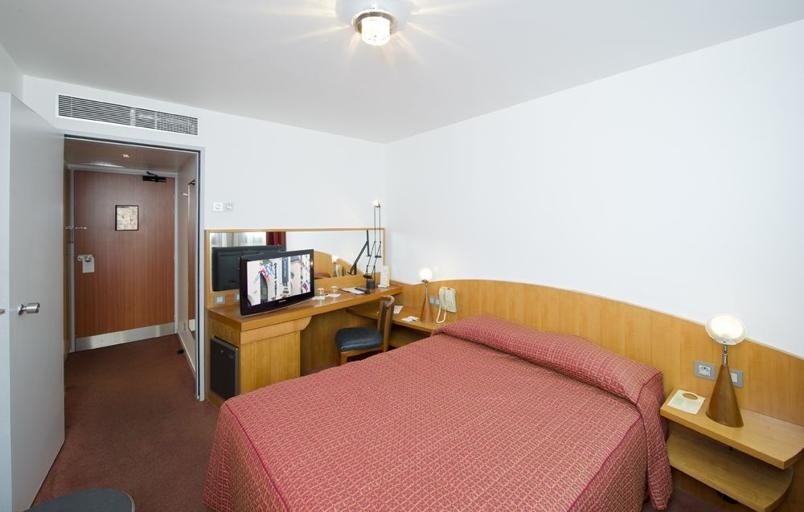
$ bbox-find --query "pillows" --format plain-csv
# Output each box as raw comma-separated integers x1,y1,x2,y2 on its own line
429,313,675,512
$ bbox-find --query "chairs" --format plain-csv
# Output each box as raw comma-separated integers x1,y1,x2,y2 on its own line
335,295,395,366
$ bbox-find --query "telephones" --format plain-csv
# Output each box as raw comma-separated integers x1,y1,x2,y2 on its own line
439,286,457,314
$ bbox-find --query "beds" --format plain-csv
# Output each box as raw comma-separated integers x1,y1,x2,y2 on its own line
200,333,648,512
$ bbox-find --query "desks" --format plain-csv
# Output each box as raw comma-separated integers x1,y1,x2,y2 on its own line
204,283,404,411
212,272,381,309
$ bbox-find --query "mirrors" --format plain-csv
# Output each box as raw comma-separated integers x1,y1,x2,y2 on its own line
203,228,386,294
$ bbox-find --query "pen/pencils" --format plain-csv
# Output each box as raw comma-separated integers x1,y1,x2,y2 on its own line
355,288,366,292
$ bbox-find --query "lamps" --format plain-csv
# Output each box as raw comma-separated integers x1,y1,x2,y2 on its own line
351,0,400,47
419,269,433,320
363,199,384,293
704,311,744,428
331,255,339,277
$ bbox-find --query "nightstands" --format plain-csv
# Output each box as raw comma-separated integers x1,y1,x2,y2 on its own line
388,308,450,348
659,388,804,512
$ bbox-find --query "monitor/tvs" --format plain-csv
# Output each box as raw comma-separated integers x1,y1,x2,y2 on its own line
211,245,284,291
240,249,314,318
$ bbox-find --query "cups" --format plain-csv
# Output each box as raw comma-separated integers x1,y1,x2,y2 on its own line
329,286,338,297
316,288,325,300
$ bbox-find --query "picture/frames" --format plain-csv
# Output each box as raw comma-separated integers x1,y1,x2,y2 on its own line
115,205,139,231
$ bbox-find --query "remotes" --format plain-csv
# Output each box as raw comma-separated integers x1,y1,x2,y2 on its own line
355,287,370,294
